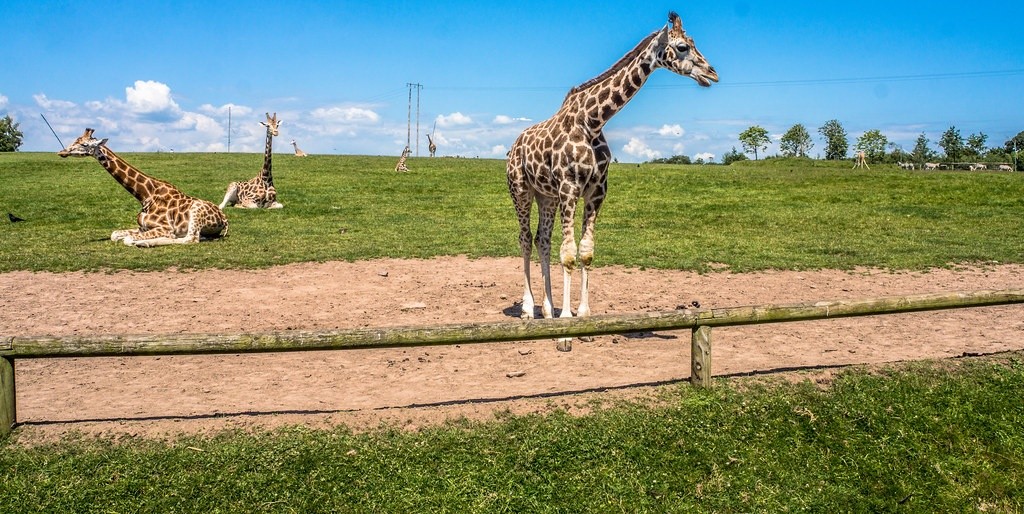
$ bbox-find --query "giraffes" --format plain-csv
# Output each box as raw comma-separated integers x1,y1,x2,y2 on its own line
217,112,284,210
395,146,412,172
505,11,717,348
426,133,436,157
290,140,307,157
58,128,229,247
851,150,870,169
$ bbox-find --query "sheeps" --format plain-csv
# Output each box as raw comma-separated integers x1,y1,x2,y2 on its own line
898,161,1013,172
